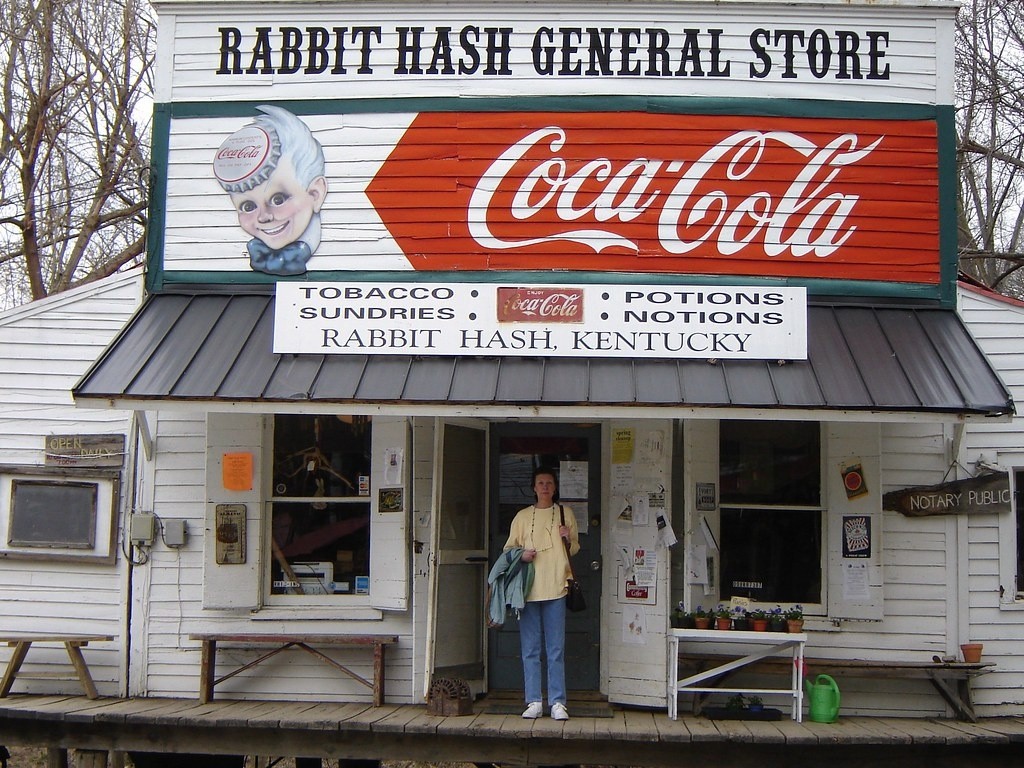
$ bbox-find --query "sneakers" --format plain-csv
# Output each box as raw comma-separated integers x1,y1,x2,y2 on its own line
521,702,543,718
550,701,569,719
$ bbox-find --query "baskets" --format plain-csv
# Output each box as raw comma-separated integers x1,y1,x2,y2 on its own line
427,676,475,717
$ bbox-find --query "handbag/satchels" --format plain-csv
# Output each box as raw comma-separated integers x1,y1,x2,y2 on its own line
567,575,583,606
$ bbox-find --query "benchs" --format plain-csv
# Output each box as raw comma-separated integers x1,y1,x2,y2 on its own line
188,629,403,707
0,635,116,700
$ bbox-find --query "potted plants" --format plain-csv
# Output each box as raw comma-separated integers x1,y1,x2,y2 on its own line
726,693,748,712
748,694,763,710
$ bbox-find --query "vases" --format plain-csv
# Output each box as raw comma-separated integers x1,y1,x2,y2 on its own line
786,618,804,633
717,617,732,629
961,643,983,663
670,616,680,629
679,616,692,629
771,621,784,631
752,618,768,632
733,619,749,630
695,618,708,629
707,618,715,629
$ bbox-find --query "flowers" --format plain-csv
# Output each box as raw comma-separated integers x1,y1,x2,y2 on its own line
669,600,806,625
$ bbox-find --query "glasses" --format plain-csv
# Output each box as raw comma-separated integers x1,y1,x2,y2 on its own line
531,528,554,553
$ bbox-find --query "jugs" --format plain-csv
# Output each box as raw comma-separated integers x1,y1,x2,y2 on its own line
805,674,842,724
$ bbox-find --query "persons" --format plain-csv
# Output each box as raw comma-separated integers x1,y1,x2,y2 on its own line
502,468,581,718
